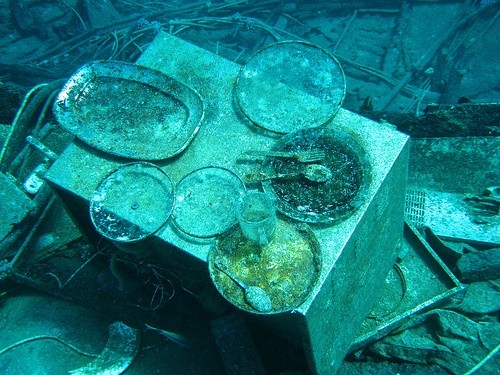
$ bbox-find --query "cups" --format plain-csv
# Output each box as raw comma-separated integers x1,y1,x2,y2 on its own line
237,192,278,247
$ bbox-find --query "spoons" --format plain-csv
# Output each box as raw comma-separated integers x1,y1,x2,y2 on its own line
242,163,333,183
213,261,272,312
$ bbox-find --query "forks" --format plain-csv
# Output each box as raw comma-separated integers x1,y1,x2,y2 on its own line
243,149,326,162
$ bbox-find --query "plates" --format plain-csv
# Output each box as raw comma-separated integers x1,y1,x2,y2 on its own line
206,215,323,317
260,127,374,223
169,168,247,239
51,59,207,162
89,163,175,244
235,39,347,134
